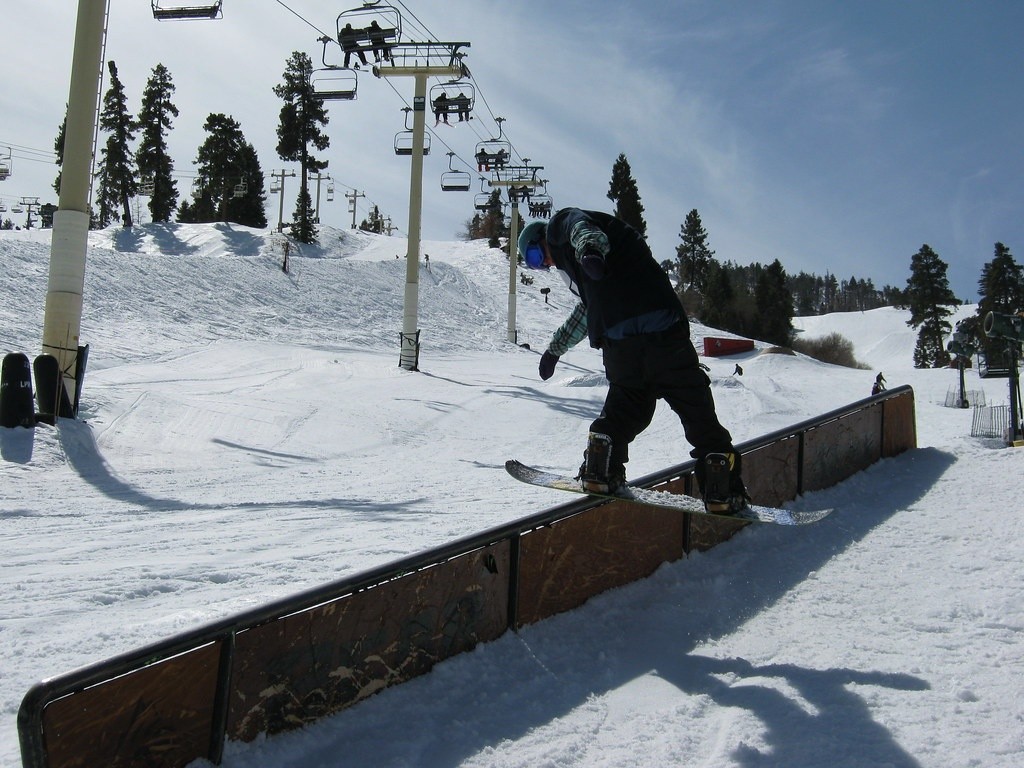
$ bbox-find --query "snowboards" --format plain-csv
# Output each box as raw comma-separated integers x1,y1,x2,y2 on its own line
503,458,835,529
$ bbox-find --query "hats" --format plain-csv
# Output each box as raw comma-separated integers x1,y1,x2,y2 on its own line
518,220,548,261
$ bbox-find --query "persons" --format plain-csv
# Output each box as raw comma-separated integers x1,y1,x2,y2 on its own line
474,148,491,172
508,184,517,203
516,206,756,517
365,19,391,63
527,200,551,219
494,148,505,172
518,185,530,204
728,359,889,397
455,92,470,122
432,91,451,125
338,22,369,70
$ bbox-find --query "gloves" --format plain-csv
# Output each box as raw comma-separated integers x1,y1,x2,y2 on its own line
582,248,612,282
540,350,559,381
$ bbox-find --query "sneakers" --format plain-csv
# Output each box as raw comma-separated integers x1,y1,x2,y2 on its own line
577,431,625,493
691,445,752,513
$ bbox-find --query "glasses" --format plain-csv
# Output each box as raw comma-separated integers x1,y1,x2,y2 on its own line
525,242,551,272
